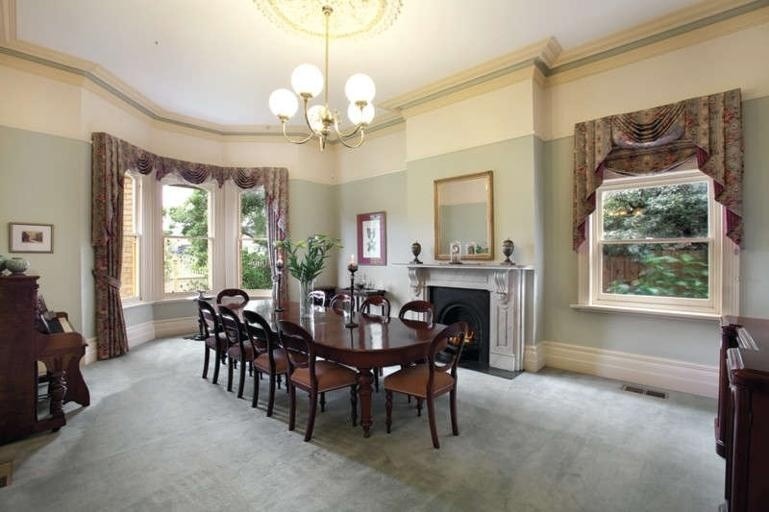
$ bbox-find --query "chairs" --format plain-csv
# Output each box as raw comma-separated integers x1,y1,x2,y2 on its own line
397,300,438,419
217,304,262,400
197,299,238,385
385,321,469,447
217,288,391,394
243,308,311,417
274,322,360,441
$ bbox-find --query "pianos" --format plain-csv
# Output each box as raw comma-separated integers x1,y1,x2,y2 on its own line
1,275,88,447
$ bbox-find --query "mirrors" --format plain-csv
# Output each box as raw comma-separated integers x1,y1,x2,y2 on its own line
432,170,493,263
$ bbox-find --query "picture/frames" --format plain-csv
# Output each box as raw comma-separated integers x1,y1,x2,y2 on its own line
7,220,54,256
355,211,386,267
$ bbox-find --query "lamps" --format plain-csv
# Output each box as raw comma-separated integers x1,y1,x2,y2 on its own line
262,6,380,156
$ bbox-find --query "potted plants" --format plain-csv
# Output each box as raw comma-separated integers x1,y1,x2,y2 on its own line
271,229,345,319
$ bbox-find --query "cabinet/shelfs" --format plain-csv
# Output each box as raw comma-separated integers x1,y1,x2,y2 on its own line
714,316,768,511
